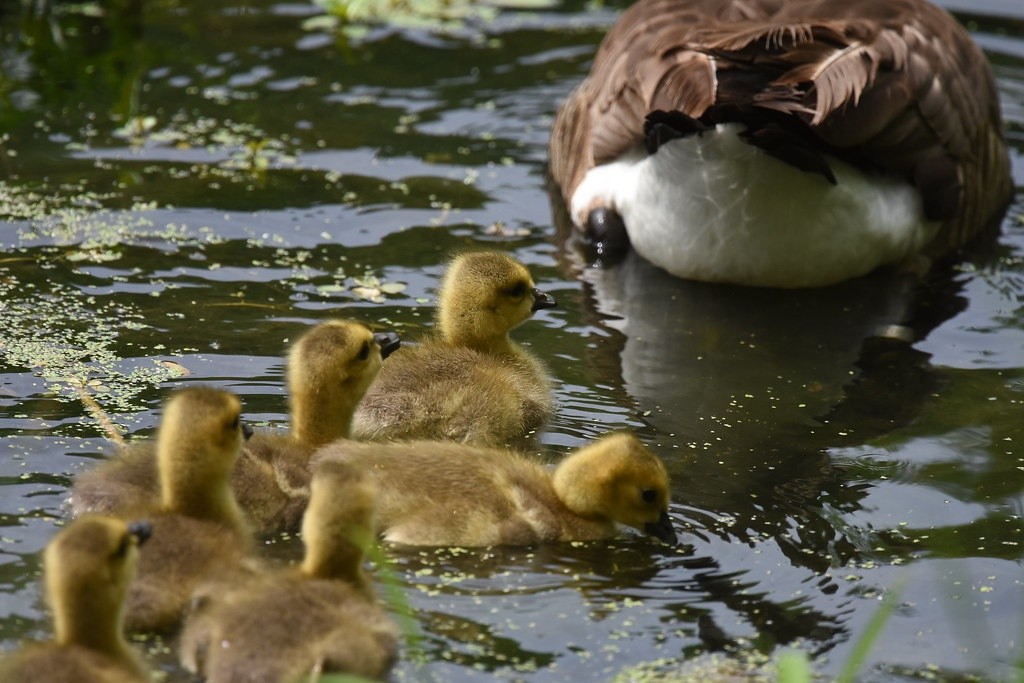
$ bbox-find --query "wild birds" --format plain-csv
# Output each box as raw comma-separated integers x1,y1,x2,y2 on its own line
547,0,1013,290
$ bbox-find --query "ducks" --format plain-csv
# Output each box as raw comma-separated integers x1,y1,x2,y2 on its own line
0,246,683,683
